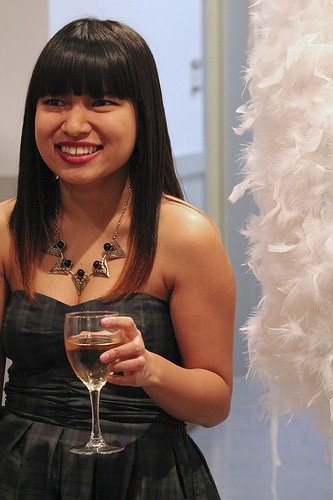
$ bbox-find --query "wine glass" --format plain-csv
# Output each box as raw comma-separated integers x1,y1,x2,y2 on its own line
65,310,125,455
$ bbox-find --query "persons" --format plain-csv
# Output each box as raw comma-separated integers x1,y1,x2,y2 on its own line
0,19,235,500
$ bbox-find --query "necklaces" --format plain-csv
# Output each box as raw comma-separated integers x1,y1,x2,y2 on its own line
46,182,131,296
